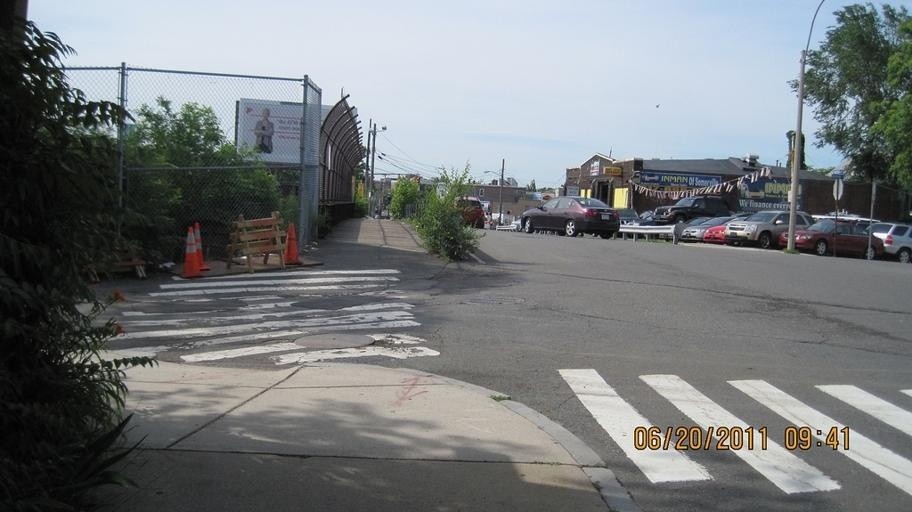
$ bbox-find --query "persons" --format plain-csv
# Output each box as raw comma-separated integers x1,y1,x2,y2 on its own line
254,106,274,153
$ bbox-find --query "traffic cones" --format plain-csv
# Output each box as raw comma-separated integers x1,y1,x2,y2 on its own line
284,222,303,265
178,221,211,279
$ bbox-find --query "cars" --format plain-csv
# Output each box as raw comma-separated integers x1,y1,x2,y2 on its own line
521,195,620,239
453,195,485,229
615,195,912,263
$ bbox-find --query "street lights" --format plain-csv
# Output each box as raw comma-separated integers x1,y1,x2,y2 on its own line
483,171,503,226
